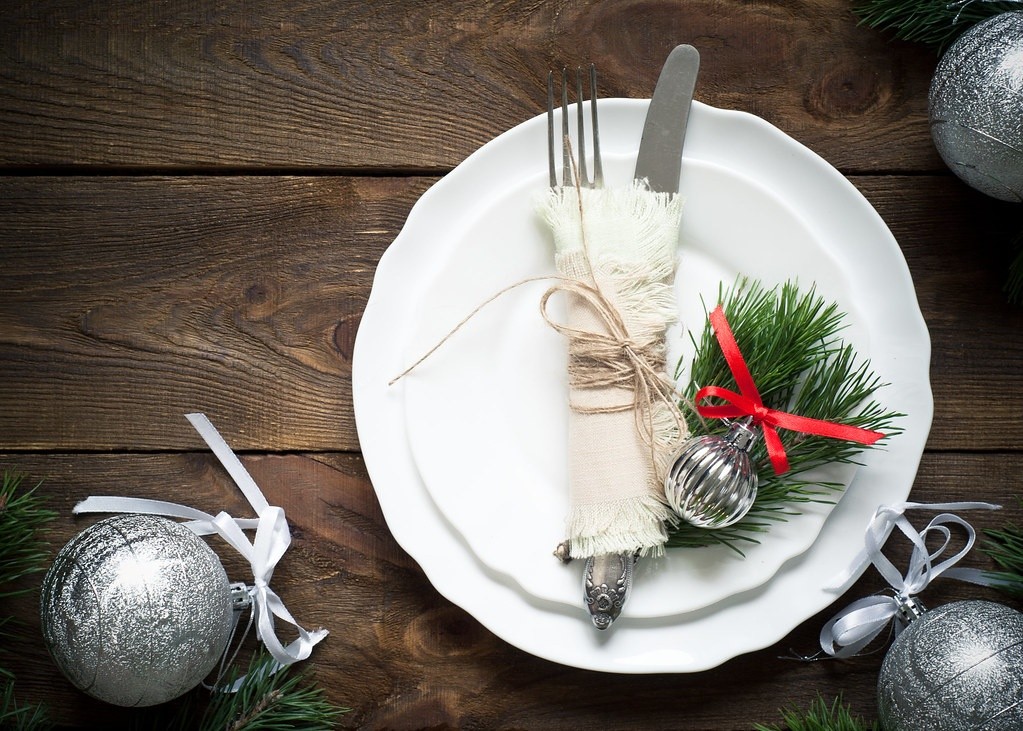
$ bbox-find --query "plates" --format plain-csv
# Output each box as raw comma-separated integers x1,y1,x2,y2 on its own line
350,93,932,685
401,155,864,623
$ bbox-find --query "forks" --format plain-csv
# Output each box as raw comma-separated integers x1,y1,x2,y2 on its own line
538,54,629,631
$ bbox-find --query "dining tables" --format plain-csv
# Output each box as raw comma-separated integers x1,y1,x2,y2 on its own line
2,2,1023,731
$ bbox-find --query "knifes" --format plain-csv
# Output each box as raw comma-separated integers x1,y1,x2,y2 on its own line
568,39,700,633
